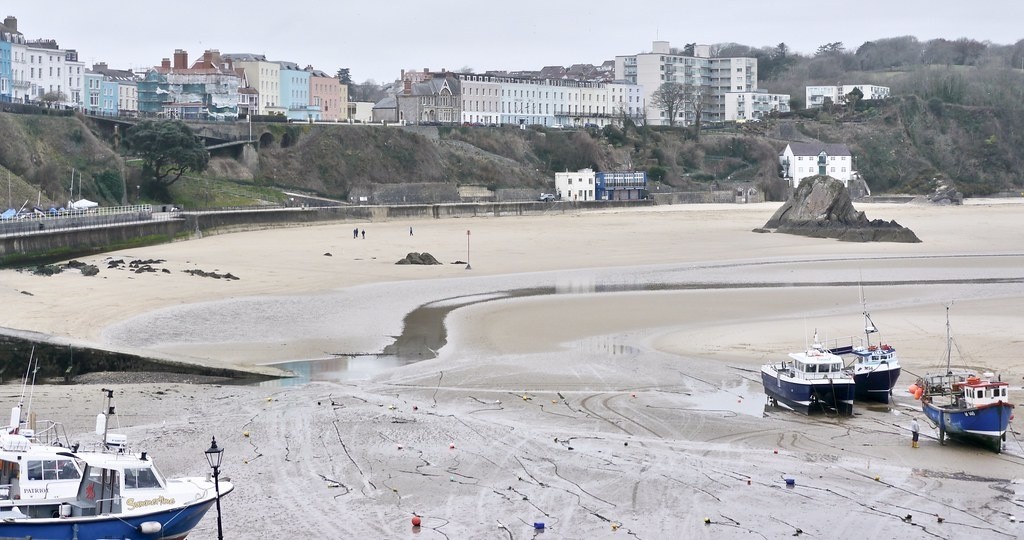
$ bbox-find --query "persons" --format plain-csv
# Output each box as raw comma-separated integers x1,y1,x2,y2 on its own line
409,226,413,236
354,227,365,240
911,417,921,448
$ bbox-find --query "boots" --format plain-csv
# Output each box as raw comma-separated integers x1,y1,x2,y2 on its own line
914,442,919,448
912,440,914,447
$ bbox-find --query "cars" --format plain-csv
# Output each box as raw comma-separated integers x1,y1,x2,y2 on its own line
551,124,564,129
488,123,497,127
538,193,555,203
472,122,485,127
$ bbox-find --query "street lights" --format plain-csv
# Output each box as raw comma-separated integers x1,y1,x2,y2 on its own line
205,434,225,539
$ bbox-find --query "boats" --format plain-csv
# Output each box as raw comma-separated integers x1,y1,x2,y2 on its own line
915,299,1014,454
760,326,856,417
815,268,901,404
0,343,236,540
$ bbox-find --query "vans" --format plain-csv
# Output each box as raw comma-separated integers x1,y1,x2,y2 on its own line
585,123,599,130
